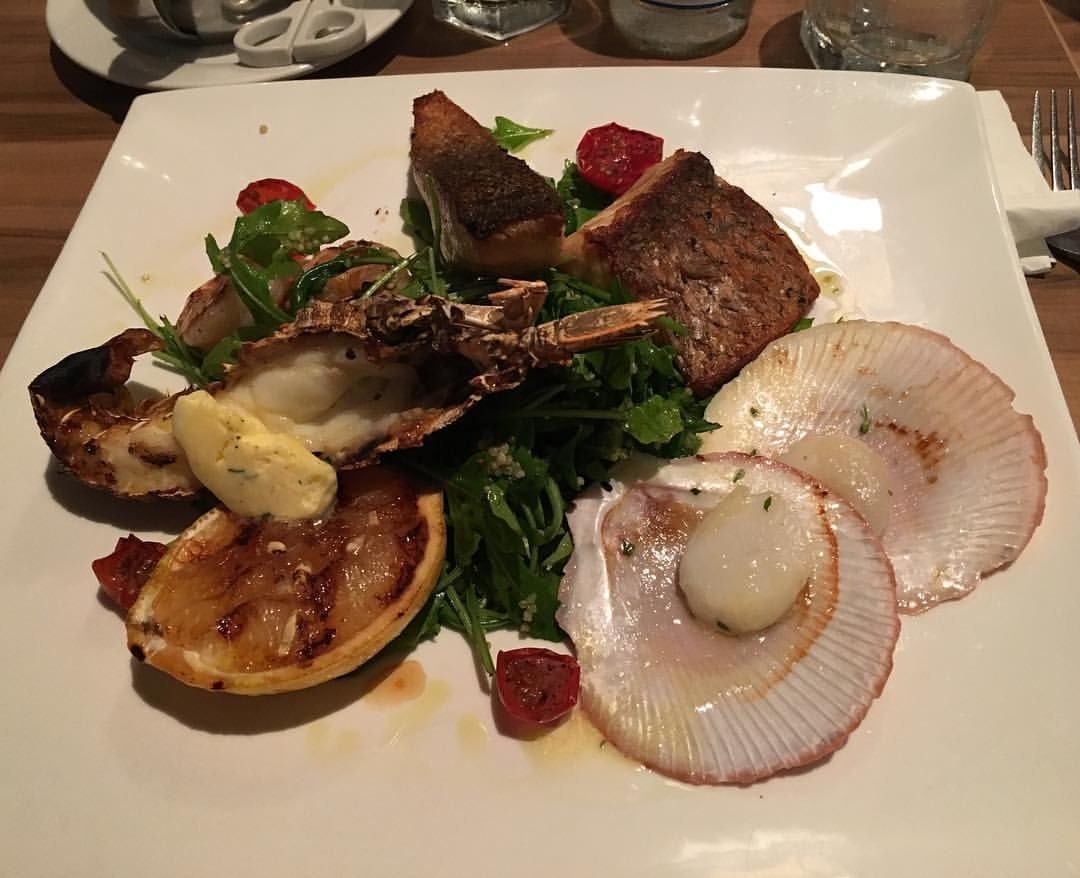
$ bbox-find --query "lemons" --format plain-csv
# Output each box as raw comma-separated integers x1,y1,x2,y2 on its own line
125,457,448,697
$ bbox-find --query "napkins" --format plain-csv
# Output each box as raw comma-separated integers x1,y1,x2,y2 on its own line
976,89,1080,274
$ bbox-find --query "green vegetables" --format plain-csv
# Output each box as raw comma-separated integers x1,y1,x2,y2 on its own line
96,115,816,676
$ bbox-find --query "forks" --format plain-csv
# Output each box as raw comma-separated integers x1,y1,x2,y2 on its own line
1031,88,1080,259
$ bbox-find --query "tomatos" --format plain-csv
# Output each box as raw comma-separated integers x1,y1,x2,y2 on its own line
236,179,317,214
494,647,583,724
575,122,665,192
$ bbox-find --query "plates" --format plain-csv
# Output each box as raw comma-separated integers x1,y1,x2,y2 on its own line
1,67,1080,878
45,0,416,90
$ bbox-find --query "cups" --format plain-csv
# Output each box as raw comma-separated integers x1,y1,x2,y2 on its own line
610,0,754,60
799,0,995,82
430,0,571,41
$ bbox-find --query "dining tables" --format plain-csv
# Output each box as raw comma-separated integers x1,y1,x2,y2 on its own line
0,0,1080,878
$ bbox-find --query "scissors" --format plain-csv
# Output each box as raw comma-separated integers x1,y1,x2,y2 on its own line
233,0,366,68
